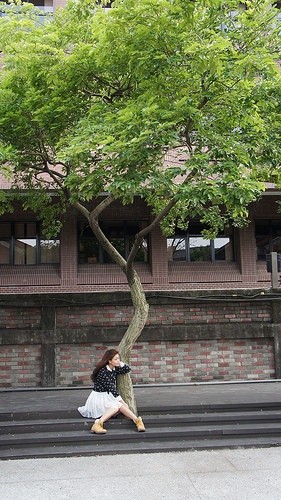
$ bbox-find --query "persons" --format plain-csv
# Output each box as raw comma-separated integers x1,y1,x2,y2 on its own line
78,349,147,434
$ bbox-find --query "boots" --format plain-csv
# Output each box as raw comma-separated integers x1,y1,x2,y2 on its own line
134,416,145,431
91,419,107,433
